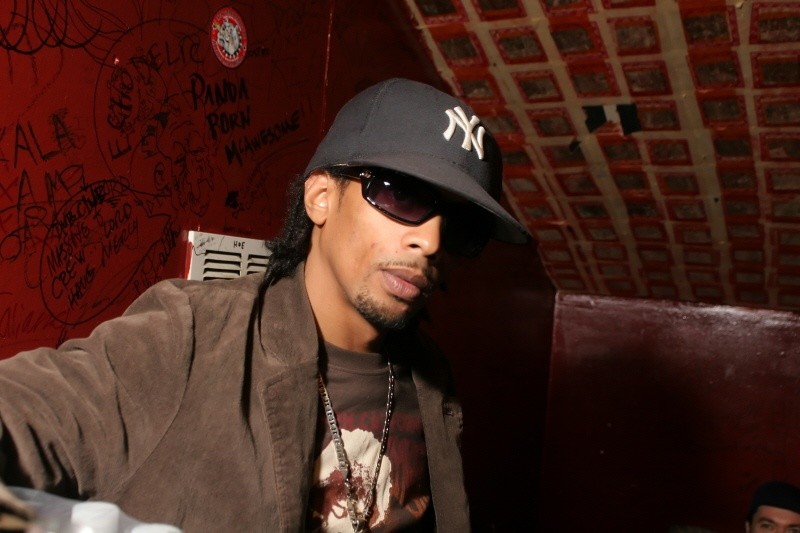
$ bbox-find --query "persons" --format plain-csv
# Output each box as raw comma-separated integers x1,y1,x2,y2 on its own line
746,480,800,533
0,77,520,533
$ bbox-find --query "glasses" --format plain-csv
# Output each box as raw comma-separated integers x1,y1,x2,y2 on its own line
326,167,490,258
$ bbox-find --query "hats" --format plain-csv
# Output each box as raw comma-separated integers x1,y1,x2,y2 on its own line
750,480,800,512
304,77,533,246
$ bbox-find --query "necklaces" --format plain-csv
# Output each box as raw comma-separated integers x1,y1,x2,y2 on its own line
317,357,390,533
